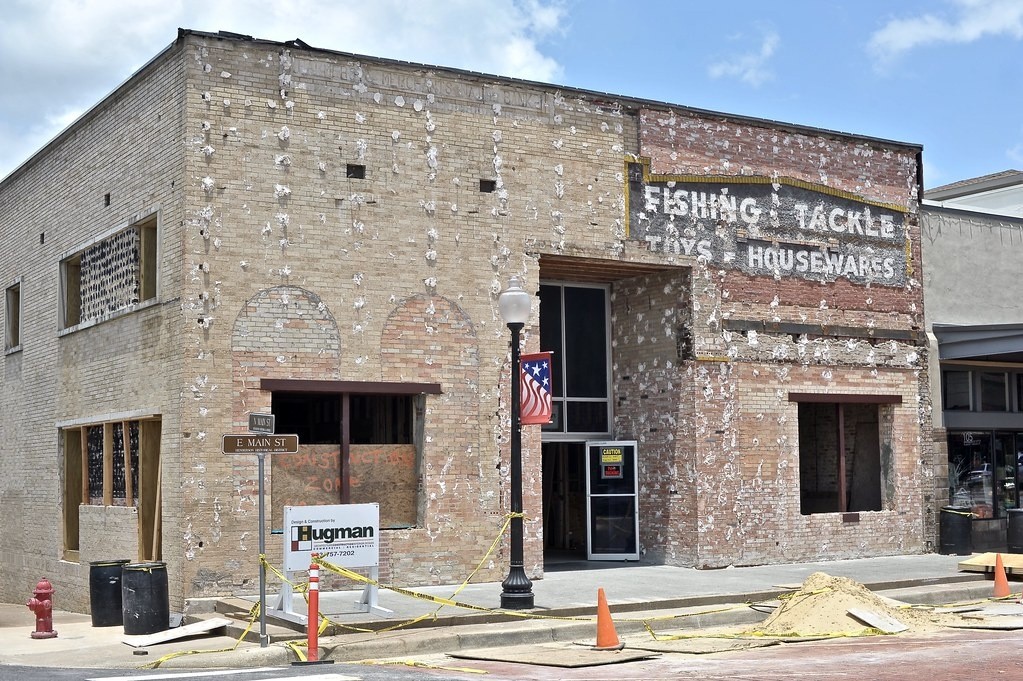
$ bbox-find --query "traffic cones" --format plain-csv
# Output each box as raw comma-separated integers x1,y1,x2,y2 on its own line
590,586,628,650
988,551,1017,601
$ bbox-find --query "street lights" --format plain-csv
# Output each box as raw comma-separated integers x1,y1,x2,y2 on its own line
499,275,536,610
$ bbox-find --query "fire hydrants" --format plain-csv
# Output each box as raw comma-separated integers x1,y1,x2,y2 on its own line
26,576,60,639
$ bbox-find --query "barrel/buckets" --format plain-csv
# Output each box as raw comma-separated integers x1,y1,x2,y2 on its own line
121,562,170,634
89,559,132,627
1006,509,1023,554
940,505,973,555
